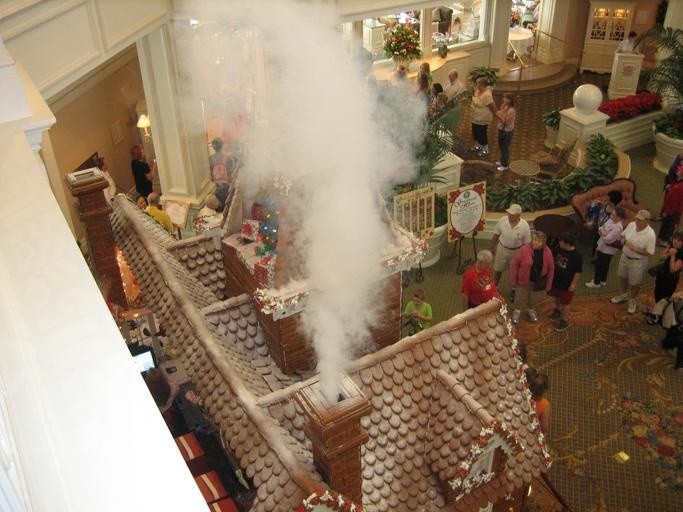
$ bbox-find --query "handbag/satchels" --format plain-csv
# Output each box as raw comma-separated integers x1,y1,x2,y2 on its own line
653,298,678,330
648,264,658,277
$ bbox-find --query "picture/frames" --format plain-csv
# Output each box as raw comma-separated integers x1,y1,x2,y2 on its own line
73,151,98,171
109,118,125,152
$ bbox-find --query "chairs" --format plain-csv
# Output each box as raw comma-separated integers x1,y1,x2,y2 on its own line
518,0,541,28
529,136,579,180
459,161,495,190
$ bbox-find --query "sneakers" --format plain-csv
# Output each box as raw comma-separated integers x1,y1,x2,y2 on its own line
511,308,521,324
585,279,607,288
610,294,629,304
553,319,571,333
469,145,489,157
550,308,561,321
527,308,538,322
627,299,637,315
496,162,509,171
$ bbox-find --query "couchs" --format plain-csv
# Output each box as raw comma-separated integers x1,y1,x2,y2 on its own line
571,177,648,258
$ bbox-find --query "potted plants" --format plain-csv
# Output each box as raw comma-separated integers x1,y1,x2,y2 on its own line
631,23,683,175
462,132,630,241
540,105,564,149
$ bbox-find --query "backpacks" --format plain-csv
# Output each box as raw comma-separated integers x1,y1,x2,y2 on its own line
477,276,497,302
210,154,229,180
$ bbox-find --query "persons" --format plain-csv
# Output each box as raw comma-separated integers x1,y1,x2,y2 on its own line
403,290,434,337
615,31,639,54
460,163,683,371
385,50,515,170
127,135,237,231
525,374,552,498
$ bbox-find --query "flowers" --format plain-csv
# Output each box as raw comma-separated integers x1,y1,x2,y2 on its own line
380,22,423,63
598,90,663,123
432,31,459,48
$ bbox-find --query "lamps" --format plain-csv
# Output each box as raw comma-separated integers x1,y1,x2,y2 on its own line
136,114,151,137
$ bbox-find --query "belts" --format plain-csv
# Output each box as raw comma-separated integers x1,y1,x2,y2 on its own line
500,240,525,250
627,254,643,261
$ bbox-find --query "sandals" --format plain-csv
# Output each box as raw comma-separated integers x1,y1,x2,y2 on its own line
643,308,659,325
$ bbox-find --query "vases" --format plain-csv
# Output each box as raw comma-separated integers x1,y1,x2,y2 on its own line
439,45,447,58
397,60,409,72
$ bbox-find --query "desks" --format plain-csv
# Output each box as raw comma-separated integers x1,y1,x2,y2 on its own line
411,147,464,196
508,27,534,67
448,7,464,31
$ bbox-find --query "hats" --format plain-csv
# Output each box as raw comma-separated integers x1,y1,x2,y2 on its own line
505,204,522,215
633,209,651,221
